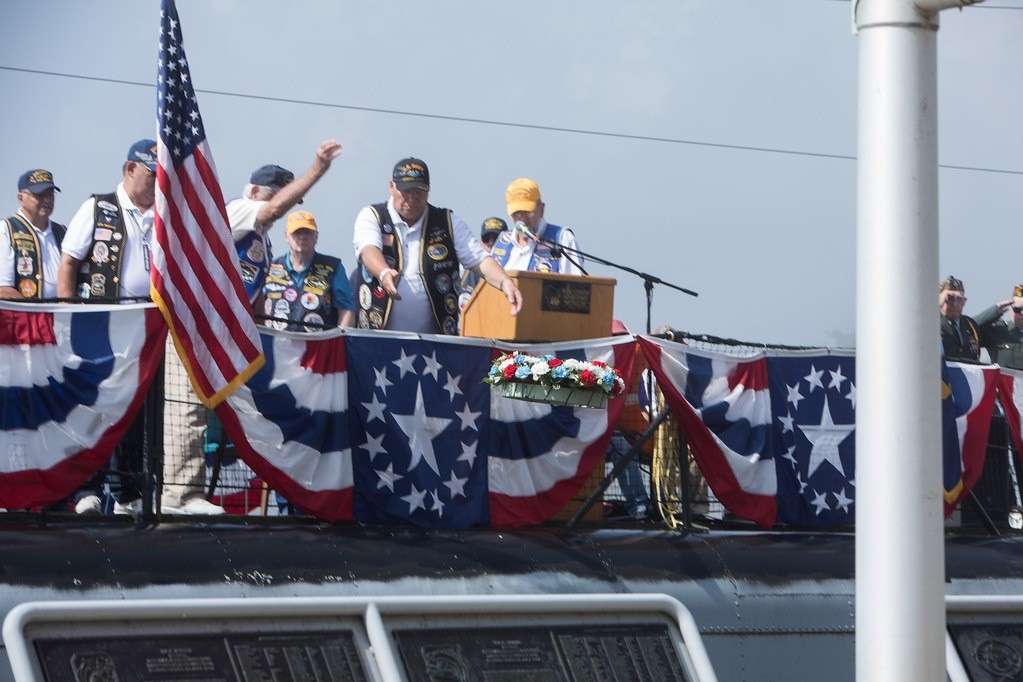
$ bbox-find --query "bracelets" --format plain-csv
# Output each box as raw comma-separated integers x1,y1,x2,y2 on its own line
500,277,514,290
378,269,390,287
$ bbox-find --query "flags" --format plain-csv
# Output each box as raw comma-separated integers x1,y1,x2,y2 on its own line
150,0,266,514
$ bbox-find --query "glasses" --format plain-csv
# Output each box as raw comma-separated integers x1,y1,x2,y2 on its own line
482,234,498,244
21,189,55,200
139,161,157,177
1010,304,1023,313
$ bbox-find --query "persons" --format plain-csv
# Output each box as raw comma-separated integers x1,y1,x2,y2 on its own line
264,211,356,333
225,140,342,325
353,158,522,336
973,285,1023,371
0,169,67,299
490,177,585,276
461,217,508,292
939,276,980,360
57,140,157,514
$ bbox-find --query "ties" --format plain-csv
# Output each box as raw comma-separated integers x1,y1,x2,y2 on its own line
949,319,962,345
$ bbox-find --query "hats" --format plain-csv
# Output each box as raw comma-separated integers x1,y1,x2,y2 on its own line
250,165,303,204
504,177,541,215
392,157,429,193
127,140,158,173
482,217,508,237
1013,284,1023,297
18,170,61,196
939,276,964,293
286,211,317,235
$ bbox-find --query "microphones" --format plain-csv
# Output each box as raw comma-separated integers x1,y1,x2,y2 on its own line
515,220,541,244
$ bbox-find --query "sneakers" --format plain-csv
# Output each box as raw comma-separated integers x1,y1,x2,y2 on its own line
112,498,163,515
75,494,104,514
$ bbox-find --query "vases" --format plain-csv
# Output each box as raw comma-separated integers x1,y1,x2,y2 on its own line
503,381,617,408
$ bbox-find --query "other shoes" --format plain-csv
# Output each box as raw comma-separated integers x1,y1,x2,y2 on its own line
162,496,226,515
627,499,655,519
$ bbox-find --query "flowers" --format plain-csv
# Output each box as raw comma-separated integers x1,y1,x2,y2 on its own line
485,353,625,391
